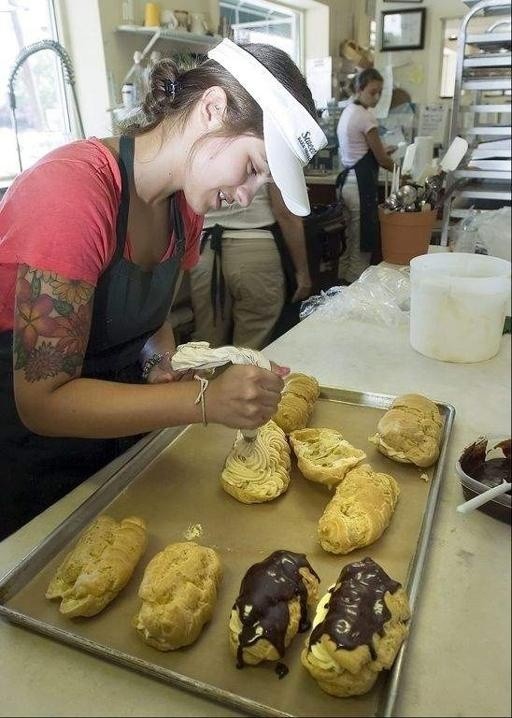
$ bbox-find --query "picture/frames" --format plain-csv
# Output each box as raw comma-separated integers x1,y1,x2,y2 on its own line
381,6,429,52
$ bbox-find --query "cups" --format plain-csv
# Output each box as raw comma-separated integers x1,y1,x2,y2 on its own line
376,203,437,266
191,13,210,35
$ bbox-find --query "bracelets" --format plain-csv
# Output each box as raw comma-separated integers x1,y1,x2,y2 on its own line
193,375,211,427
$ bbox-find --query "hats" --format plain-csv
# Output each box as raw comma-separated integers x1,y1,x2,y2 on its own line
207,37,329,217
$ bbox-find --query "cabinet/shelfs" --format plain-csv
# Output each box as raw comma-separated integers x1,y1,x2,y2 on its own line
438,3,512,243
263,170,349,213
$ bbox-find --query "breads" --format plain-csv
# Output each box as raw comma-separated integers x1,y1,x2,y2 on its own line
270,373,320,435
288,428,368,491
369,394,445,468
45,516,146,620
317,465,401,554
229,549,321,679
131,541,220,651
300,556,411,699
220,420,291,506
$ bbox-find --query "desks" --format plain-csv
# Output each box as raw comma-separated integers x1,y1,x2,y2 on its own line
0,260,510,716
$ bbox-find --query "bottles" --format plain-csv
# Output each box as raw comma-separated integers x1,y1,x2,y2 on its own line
121,82,135,106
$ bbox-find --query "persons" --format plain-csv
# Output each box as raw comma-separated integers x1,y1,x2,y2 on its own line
334,64,404,286
186,179,313,352
2,39,317,527
